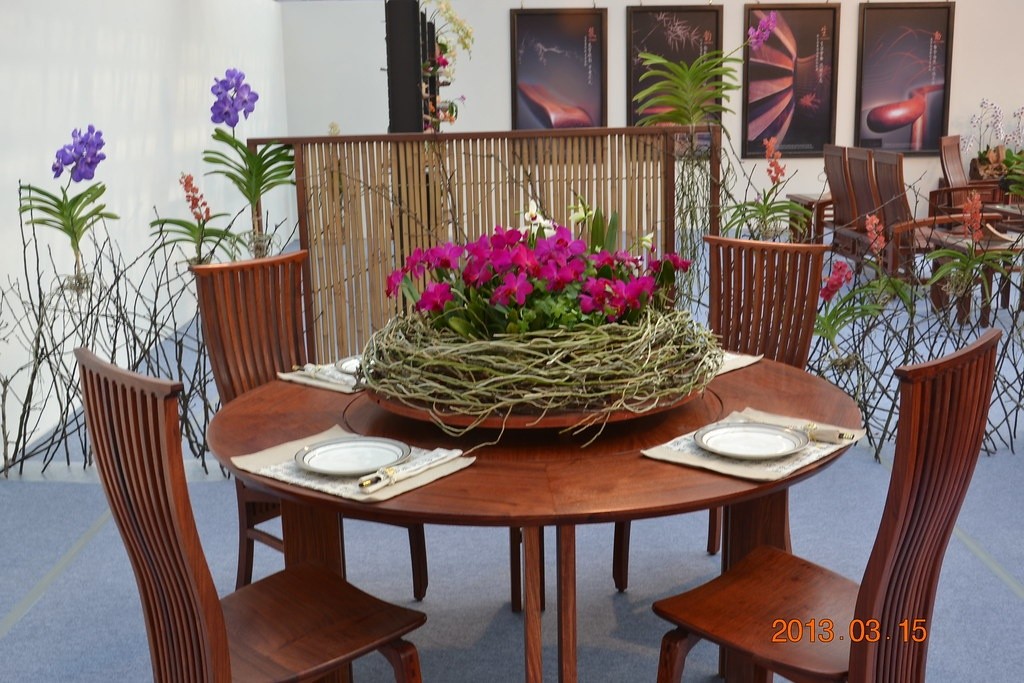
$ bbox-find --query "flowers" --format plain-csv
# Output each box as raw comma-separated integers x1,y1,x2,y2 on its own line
421,0,476,133
384,186,688,321
18,67,301,288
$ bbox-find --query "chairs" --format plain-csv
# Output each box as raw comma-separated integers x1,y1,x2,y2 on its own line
189,248,430,604
929,135,1005,218
604,235,834,595
653,329,1006,683
74,348,427,683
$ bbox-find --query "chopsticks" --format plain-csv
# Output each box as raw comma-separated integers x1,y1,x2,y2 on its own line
291,365,305,372
358,477,380,487
838,433,854,441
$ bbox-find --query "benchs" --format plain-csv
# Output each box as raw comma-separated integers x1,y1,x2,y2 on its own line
823,143,1000,297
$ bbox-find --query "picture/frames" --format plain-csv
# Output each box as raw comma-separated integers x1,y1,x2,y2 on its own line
510,7,611,166
741,3,841,159
626,6,724,164
855,2,957,152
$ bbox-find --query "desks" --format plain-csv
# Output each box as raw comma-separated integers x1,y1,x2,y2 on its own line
926,234,1024,326
206,350,863,683
981,204,1024,242
786,194,833,246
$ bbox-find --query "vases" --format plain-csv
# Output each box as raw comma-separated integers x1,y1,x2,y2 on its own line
349,320,721,456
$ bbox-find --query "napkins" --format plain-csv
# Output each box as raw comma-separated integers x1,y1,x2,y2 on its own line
754,413,842,446
303,360,366,387
357,447,463,493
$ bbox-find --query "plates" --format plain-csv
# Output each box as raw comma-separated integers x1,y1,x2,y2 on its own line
694,422,811,459
295,435,410,475
335,355,374,378
